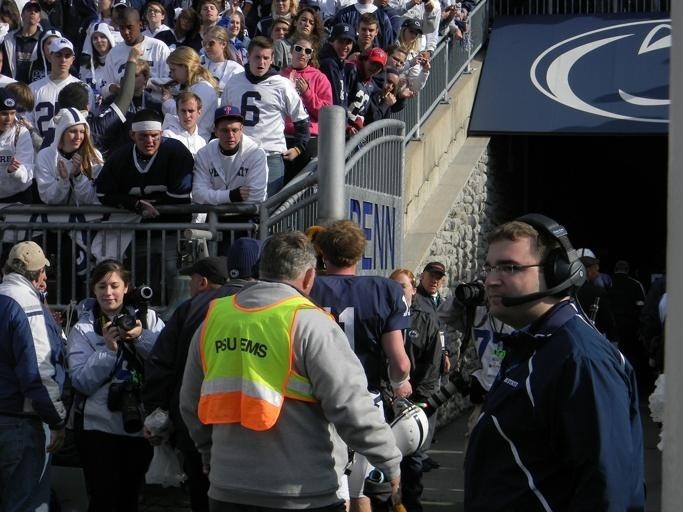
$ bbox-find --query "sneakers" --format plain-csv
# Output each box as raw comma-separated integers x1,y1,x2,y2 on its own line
421,457,441,472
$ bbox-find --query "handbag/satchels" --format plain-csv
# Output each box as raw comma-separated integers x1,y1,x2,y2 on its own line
50,390,85,469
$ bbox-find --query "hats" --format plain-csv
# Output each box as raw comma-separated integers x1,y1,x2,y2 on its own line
5,240,51,272
178,256,228,284
227,237,260,275
334,19,422,67
0,86,18,111
425,260,447,274
108,0,132,11
612,259,630,275
214,105,245,120
574,246,599,267
49,36,74,53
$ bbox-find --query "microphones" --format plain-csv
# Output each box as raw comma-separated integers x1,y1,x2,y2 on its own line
502,280,572,308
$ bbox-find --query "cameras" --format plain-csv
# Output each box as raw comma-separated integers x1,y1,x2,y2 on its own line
111,312,136,331
423,372,470,419
108,382,143,433
455,280,488,306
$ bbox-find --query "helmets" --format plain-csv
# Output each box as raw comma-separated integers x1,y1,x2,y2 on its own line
385,401,430,454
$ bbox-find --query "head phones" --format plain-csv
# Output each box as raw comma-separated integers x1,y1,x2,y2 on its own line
514,213,587,298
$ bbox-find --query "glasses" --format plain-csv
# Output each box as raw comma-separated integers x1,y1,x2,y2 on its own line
480,262,541,275
294,43,314,55
147,10,162,13
